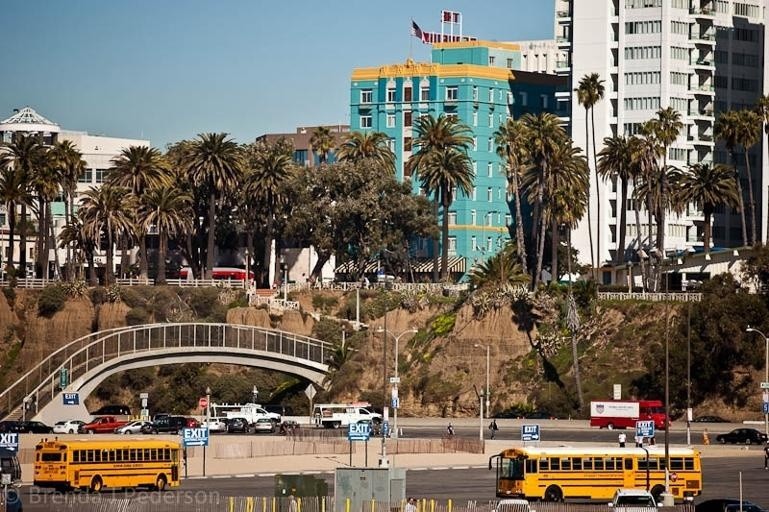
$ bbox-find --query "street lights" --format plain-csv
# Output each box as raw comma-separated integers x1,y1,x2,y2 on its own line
251,382,259,404
282,263,288,310
354,280,362,330
244,247,250,290
204,386,213,434
472,342,494,417
746,326,769,438
374,326,418,438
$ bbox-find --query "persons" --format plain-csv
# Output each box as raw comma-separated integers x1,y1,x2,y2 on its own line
763,440,769,470
618,431,656,447
702,427,710,445
488,418,500,440
446,421,456,437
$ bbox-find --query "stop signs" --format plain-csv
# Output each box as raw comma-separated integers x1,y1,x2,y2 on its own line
199,398,207,407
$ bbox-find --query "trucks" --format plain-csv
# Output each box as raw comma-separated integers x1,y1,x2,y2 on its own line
210,402,283,425
312,403,384,428
1,446,23,504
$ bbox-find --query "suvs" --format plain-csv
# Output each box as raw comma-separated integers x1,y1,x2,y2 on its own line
606,488,665,512
90,404,132,416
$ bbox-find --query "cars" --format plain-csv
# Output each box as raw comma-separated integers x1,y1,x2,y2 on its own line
716,427,768,445
490,497,538,512
688,415,732,423
695,498,766,512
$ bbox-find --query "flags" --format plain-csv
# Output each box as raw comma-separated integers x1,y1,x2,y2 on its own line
411,20,426,45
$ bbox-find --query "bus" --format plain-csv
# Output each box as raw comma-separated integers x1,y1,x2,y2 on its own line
178,265,255,285
32,435,184,495
487,448,706,504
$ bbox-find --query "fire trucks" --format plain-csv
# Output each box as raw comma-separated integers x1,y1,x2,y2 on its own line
589,400,673,430
